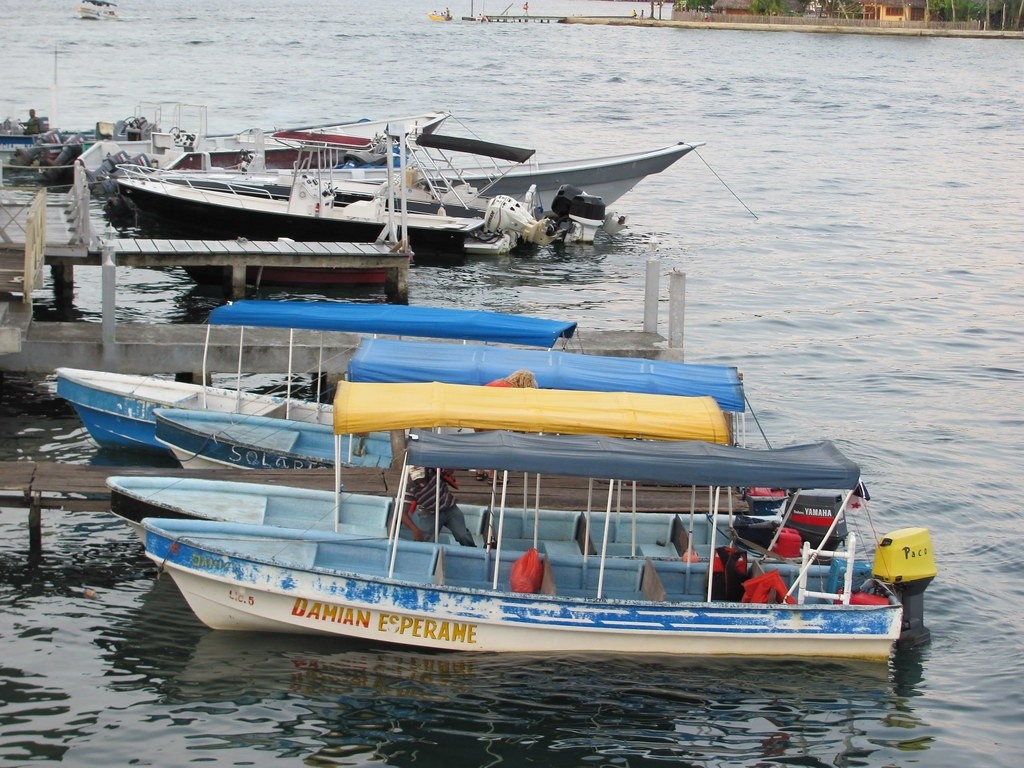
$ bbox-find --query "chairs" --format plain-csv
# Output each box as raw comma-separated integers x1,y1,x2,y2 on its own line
95,122,116,140
151,132,174,154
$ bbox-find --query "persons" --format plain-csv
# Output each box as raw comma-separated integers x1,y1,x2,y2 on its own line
523,2,529,16
239,149,254,175
432,8,450,18
401,466,477,548
632,8,644,20
19,109,43,135
474,369,539,486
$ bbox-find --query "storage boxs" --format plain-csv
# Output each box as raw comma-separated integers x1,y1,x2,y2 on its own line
827,557,871,593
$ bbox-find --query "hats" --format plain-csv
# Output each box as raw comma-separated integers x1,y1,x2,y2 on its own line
409,465,426,481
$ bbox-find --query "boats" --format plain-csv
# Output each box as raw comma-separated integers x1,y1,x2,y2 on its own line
1,110,939,668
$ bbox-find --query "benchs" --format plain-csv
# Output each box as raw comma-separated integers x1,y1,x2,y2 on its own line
263,402,287,420
448,510,490,548
498,551,557,595
352,497,396,537
605,556,666,602
543,512,597,556
393,547,445,586
361,454,393,468
639,514,693,558
351,170,364,179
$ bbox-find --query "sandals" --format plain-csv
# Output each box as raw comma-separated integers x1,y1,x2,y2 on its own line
476,472,488,481
488,476,510,485
